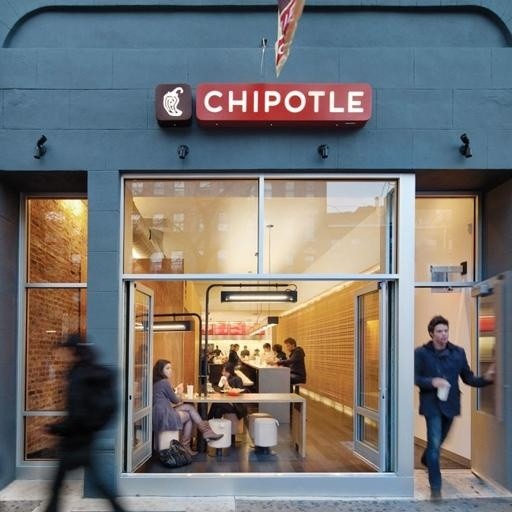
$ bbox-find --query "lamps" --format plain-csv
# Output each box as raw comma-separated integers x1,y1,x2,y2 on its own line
459,133,473,159
177,144,190,159
32,135,47,158
247,317,278,336
221,284,298,303
318,145,329,159
135,313,202,331
234,369,254,387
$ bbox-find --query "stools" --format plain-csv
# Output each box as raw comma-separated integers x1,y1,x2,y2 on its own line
291,383,306,412
153,412,279,457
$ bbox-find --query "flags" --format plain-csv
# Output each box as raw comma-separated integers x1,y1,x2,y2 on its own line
274,0,304,78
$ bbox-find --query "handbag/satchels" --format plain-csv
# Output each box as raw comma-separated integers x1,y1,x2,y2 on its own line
159,439,192,468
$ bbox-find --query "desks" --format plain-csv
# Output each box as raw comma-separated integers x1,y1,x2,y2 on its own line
175,392,307,458
241,359,291,423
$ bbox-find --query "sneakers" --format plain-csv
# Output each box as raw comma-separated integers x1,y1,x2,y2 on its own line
430,487,441,501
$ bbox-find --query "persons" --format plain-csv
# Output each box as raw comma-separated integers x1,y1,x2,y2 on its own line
46,332,125,512
207,363,248,421
203,337,306,384
153,359,225,456
414,315,496,496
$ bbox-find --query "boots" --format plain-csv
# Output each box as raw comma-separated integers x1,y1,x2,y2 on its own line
198,420,224,442
180,438,199,456
